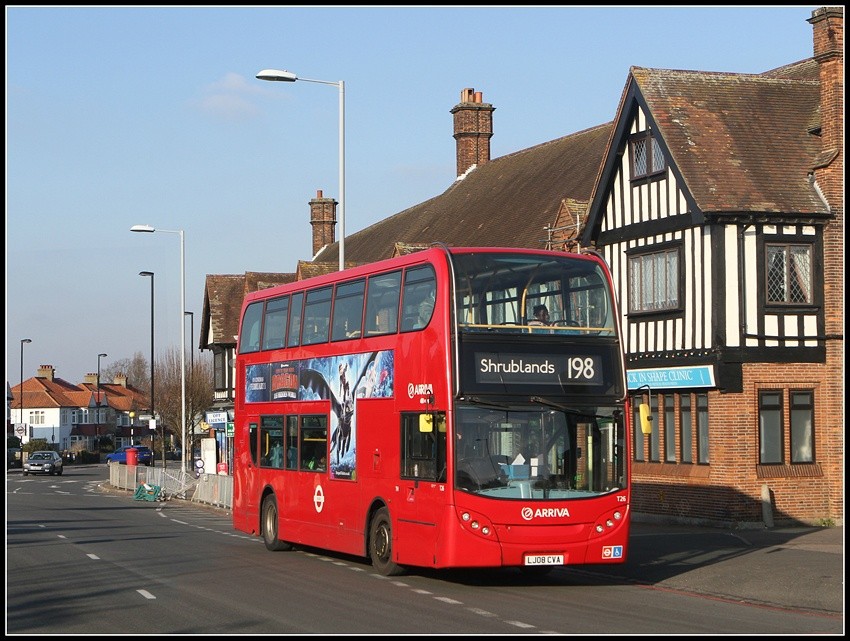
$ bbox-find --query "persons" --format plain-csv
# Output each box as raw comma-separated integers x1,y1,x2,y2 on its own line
338,362,349,401
418,286,436,323
533,305,558,326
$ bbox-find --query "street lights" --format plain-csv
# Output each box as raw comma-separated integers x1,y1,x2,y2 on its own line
254,69,347,272
19,338,32,468
129,224,187,498
129,412,135,448
184,311,195,471
96,354,108,448
139,271,155,468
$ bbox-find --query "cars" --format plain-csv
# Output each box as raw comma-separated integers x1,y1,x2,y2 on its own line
105,445,152,467
23,450,64,476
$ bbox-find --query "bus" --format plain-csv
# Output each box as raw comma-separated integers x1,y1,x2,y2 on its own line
232,242,655,577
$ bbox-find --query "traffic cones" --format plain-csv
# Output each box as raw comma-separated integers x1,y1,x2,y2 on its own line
141,481,154,494
